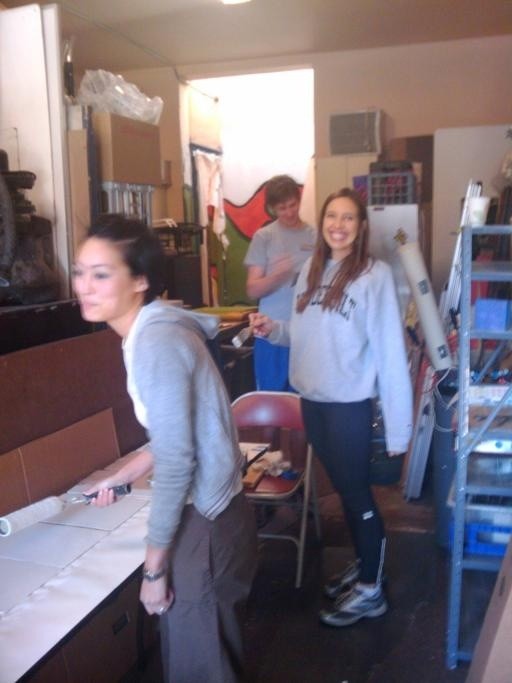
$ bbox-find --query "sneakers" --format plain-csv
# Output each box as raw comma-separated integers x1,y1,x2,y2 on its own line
314,557,388,627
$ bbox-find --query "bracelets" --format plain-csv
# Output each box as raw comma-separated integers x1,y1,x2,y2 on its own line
142,565,168,580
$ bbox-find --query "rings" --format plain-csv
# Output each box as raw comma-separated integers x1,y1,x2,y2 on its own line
159,605,165,611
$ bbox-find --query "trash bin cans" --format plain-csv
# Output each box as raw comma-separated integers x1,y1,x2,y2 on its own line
435,372,467,547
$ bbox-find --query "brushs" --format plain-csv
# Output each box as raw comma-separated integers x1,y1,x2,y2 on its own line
0,483,131,538
231,315,265,349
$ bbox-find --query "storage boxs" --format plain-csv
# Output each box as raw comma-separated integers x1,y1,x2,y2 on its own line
87,111,162,186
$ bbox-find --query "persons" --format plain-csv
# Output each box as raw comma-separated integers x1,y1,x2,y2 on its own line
71,212,259,682
243,174,320,391
246,186,416,626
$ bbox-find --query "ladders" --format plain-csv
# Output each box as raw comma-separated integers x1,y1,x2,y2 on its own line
403,178,512,670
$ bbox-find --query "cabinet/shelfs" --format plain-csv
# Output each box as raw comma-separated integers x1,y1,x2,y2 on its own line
442,224,512,672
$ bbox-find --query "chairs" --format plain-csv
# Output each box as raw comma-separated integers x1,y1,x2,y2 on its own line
231,391,322,590
